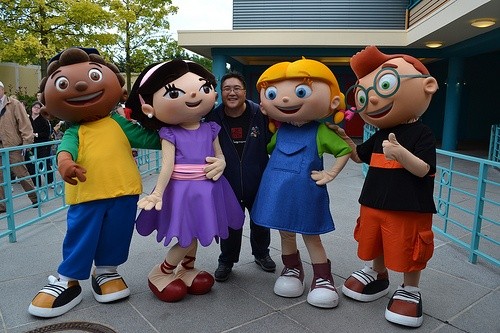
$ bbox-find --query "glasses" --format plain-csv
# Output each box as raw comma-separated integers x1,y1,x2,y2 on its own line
221,87,246,93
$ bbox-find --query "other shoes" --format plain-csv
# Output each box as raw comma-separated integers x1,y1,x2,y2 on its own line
32,201,39,208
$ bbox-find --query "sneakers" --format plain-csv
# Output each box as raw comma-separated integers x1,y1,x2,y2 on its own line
254,255,276,271
214,264,233,282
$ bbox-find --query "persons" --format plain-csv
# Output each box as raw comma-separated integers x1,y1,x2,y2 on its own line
29,48,161,318
204,72,277,283
0,82,138,214
124,58,245,303
250,56,351,309
325,46,437,329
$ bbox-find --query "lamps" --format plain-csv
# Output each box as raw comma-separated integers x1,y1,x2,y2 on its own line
425,44,442,48
471,21,495,27
